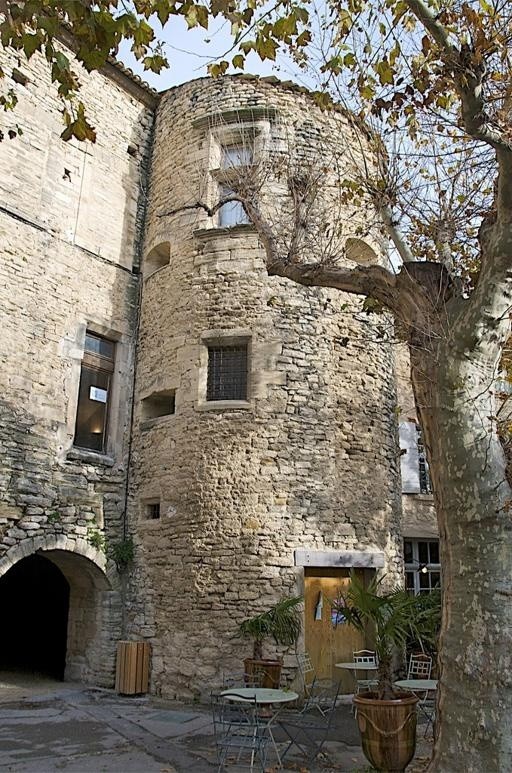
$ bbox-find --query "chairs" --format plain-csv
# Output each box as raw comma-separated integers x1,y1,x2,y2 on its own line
405,653,432,680
294,653,334,717
223,672,276,763
353,649,379,720
210,692,270,773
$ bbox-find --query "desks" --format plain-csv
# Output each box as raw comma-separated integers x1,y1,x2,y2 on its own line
220,690,299,763
333,663,380,712
393,679,438,738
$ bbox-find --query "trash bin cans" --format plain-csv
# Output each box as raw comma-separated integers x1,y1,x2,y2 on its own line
115,640,150,699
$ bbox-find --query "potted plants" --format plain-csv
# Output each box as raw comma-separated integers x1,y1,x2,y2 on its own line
324,567,440,773
111,538,136,573
225,594,305,689
